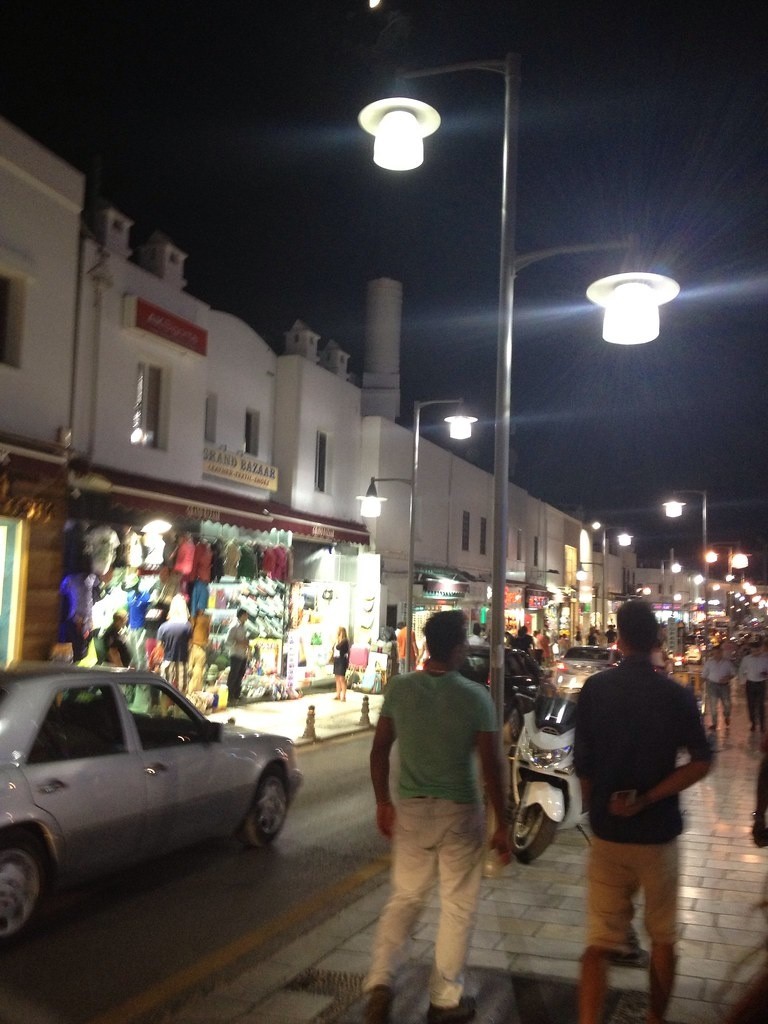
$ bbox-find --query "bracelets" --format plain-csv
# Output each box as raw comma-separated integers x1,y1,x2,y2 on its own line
377,801,392,806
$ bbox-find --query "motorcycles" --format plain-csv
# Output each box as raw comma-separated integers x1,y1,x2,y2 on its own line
513,669,591,860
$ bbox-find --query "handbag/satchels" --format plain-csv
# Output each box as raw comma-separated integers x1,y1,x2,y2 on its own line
329,642,336,665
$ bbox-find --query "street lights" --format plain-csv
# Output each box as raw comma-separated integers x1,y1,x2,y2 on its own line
688,572,702,632
358,54,679,732
591,520,635,646
705,541,748,640
643,560,681,617
661,488,708,663
354,396,476,671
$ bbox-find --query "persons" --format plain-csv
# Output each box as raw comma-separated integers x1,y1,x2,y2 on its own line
654,628,768,732
333,628,349,701
466,623,618,665
393,622,431,677
362,611,513,1024
224,609,252,707
157,595,193,717
574,600,715,1024
101,609,140,671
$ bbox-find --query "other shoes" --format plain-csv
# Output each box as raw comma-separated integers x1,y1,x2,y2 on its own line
725,715,731,726
708,724,717,730
604,950,649,967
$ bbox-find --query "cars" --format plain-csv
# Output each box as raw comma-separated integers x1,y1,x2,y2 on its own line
684,635,702,665
465,645,560,743
0,665,302,948
556,645,623,694
697,634,709,651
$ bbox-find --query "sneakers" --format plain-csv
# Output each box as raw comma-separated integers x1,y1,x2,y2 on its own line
364,985,393,1021
427,996,476,1024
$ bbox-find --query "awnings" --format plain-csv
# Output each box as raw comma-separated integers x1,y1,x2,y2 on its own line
72,463,371,548
